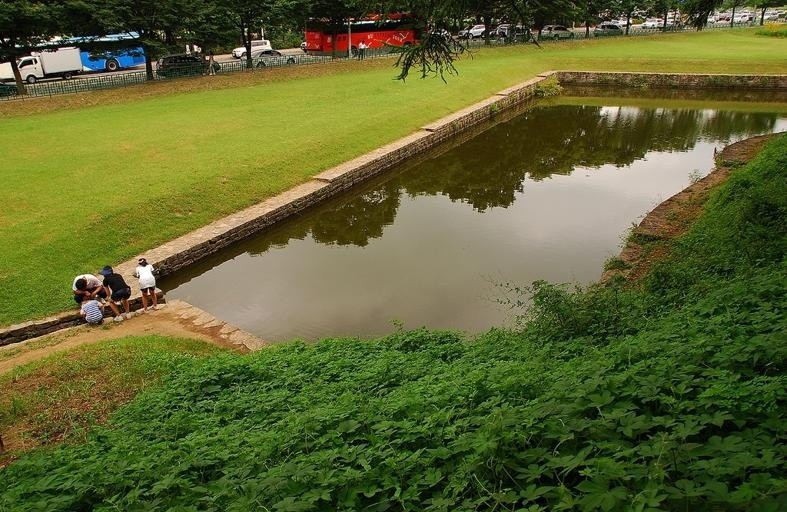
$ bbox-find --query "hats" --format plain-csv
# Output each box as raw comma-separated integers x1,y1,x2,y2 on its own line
98,270,112,275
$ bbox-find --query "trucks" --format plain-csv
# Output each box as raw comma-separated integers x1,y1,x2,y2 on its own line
14,48,83,83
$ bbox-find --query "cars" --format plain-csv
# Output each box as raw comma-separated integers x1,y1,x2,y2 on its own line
641,7,787,32
239,50,296,68
428,27,451,41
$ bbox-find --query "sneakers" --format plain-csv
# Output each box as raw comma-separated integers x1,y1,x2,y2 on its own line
125,313,132,319
151,305,158,311
140,308,147,313
98,320,104,325
114,315,123,321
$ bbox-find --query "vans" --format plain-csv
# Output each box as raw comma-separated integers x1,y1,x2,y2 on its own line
458,23,624,42
233,39,272,58
157,56,220,77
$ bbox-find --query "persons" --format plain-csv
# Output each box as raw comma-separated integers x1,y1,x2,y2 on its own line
358,39,367,61
72,273,107,305
98,265,131,322
209,51,216,76
79,296,104,325
133,258,157,312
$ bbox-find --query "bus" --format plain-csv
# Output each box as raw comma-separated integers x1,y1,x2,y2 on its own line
45,32,146,74
306,12,432,58
